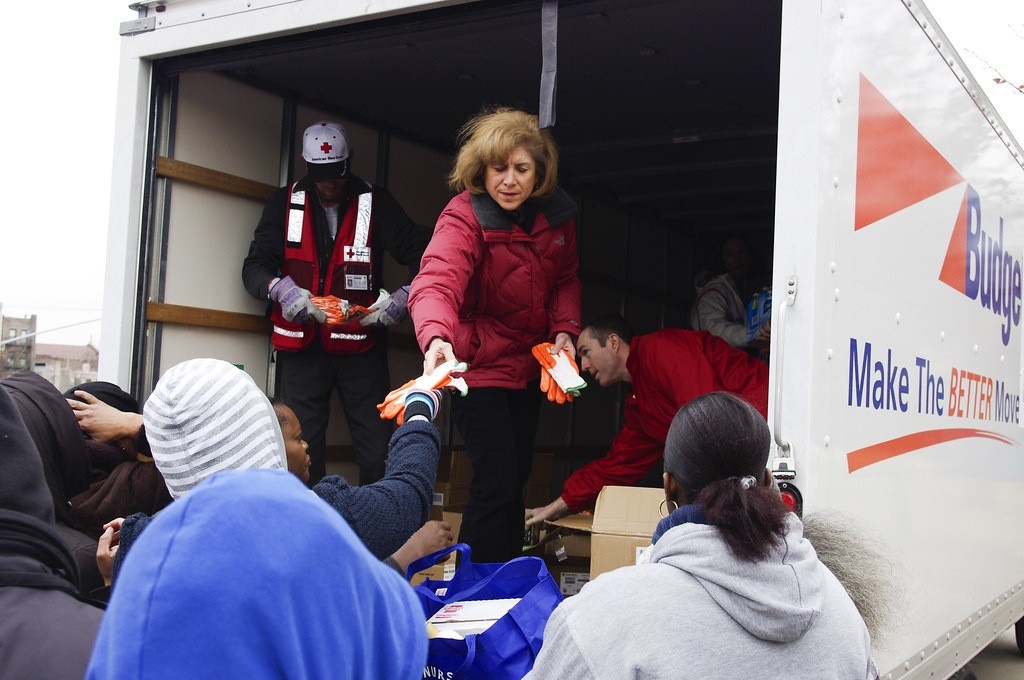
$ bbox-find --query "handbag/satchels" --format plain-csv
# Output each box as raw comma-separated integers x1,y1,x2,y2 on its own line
405,543,564,680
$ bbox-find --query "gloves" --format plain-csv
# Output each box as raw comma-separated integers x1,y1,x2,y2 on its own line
532,342,587,404
359,285,410,327
377,359,468,426
309,288,391,328
403,387,443,421
269,275,327,324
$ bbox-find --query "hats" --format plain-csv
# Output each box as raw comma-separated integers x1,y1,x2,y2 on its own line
63,382,139,440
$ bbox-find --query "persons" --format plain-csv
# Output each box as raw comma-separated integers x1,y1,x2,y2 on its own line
264,387,440,560
406,111,583,563
77,466,429,680
0,356,288,607
522,390,872,680
524,315,769,533
242,119,429,486
0,385,107,679
383,521,454,579
688,236,771,361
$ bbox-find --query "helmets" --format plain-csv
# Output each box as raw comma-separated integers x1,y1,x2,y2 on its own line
301,122,350,182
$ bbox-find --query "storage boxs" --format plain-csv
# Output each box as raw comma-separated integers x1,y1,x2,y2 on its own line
408,447,674,599
